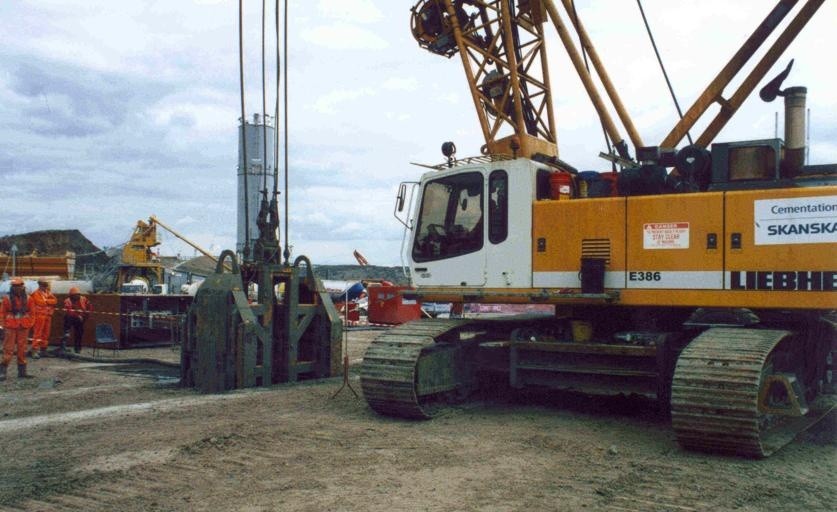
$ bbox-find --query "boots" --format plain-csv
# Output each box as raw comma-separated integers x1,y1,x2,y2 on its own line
31,347,56,359
0,363,8,380
17,362,34,378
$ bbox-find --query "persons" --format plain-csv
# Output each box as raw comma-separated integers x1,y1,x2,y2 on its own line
31,277,59,359
59,286,94,353
0,276,37,382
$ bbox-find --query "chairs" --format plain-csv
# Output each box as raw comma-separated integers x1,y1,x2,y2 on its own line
93,322,120,357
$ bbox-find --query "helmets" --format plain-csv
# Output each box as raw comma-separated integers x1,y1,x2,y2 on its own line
70,288,80,296
11,278,24,285
38,277,48,283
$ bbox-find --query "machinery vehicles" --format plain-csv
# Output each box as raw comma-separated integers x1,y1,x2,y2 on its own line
360,0,836,461
117,278,148,295
